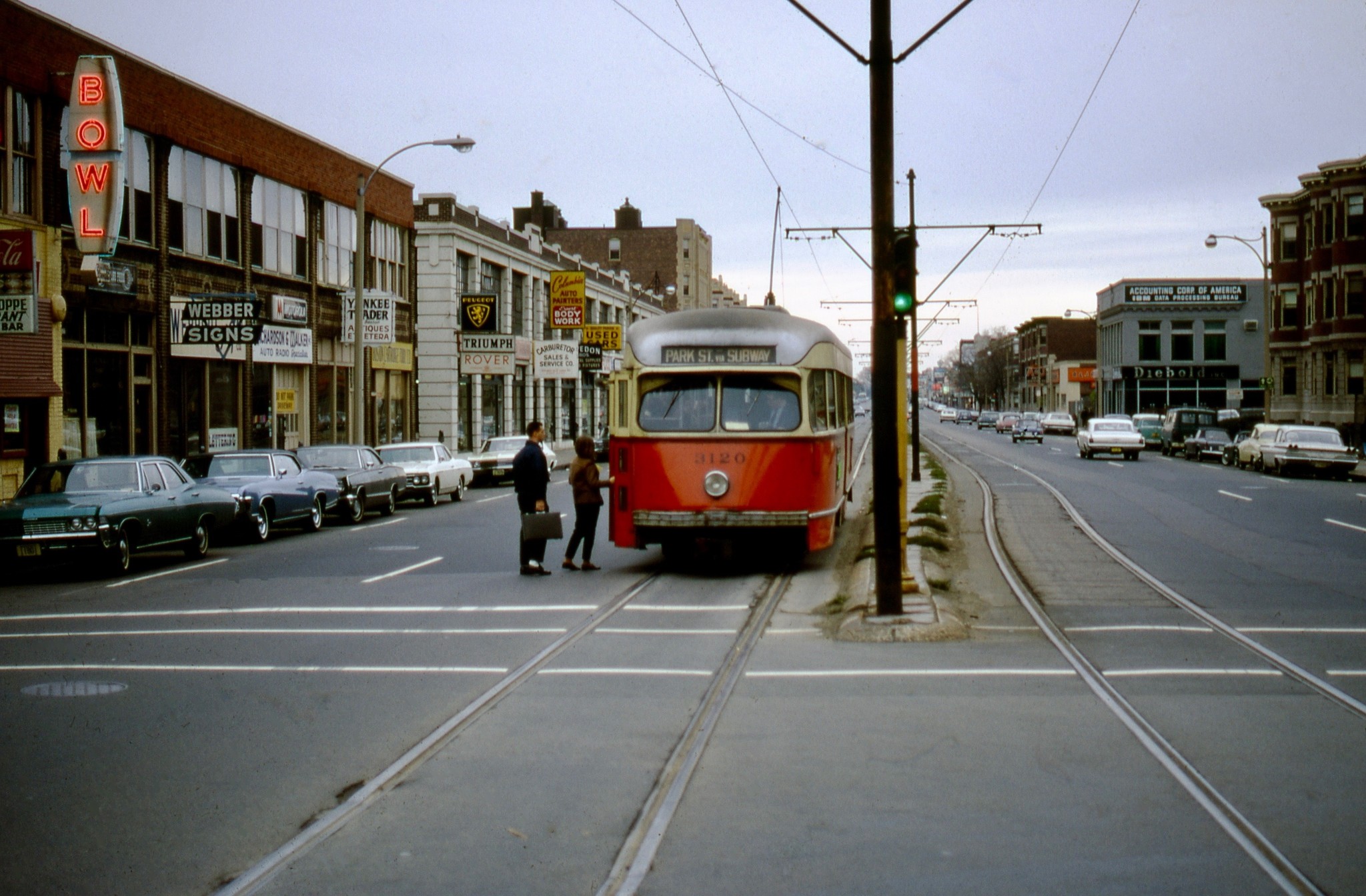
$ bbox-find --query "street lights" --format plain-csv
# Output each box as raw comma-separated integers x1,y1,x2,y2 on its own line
987,347,1010,412
350,133,476,446
1065,305,1103,419
1205,226,1270,423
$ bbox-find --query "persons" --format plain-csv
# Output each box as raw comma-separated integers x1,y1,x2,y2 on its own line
562,435,616,571
757,384,802,431
513,420,555,576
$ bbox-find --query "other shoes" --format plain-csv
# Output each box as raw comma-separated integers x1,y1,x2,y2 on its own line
519,565,537,575
532,563,551,576
560,561,580,570
581,563,601,571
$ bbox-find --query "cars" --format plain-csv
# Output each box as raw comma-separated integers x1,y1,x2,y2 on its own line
853,391,1358,480
0,421,608,580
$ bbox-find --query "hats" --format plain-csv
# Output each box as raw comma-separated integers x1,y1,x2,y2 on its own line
575,436,586,454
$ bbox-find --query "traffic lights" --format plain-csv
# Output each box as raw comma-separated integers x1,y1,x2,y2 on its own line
893,230,916,317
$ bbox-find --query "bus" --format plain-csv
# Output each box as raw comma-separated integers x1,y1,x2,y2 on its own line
607,305,855,575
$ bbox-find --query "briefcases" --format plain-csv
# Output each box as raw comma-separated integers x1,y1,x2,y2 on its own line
521,507,563,541
